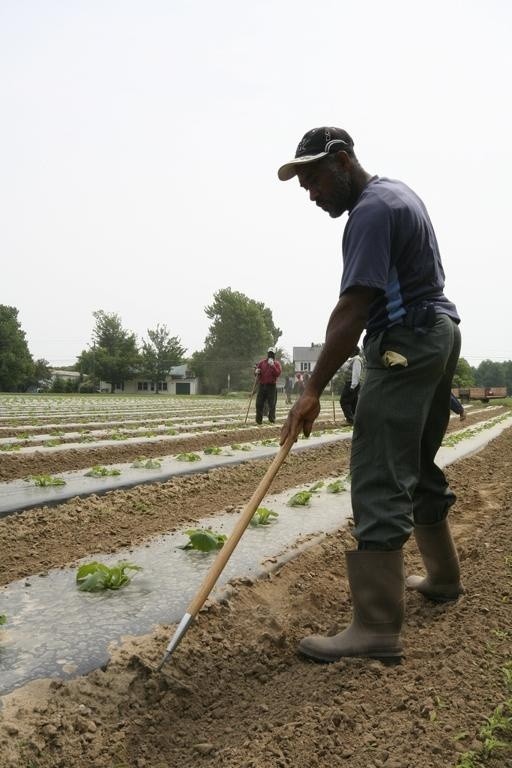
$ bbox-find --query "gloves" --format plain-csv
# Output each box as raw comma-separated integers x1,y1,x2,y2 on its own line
268,358,274,366
254,368,261,374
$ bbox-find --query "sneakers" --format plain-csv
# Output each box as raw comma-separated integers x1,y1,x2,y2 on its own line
340,421,353,426
459,410,467,422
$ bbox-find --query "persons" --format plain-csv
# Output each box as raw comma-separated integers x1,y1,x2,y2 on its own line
340,347,362,427
277,126,469,666
294,373,303,398
449,393,466,421
284,376,293,404
253,346,282,424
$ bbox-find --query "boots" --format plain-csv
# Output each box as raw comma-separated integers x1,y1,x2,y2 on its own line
405,519,466,599
298,548,405,662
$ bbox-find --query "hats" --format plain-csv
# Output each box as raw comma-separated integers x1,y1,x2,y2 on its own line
267,346,276,354
278,126,355,182
